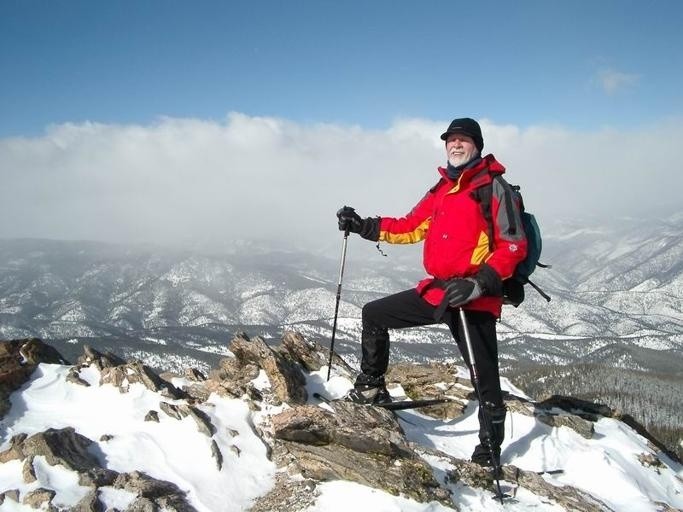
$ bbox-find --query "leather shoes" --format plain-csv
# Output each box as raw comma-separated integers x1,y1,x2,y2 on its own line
442,263,502,308
337,205,380,242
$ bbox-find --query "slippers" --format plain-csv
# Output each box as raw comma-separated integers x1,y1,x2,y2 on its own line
503,184,542,308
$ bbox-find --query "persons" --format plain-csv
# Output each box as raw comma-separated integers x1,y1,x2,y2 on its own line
333,116,531,483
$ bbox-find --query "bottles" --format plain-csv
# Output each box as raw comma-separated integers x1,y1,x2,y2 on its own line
441,117,483,152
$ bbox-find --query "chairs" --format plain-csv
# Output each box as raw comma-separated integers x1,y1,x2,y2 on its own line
330,373,392,406
472,438,500,466
